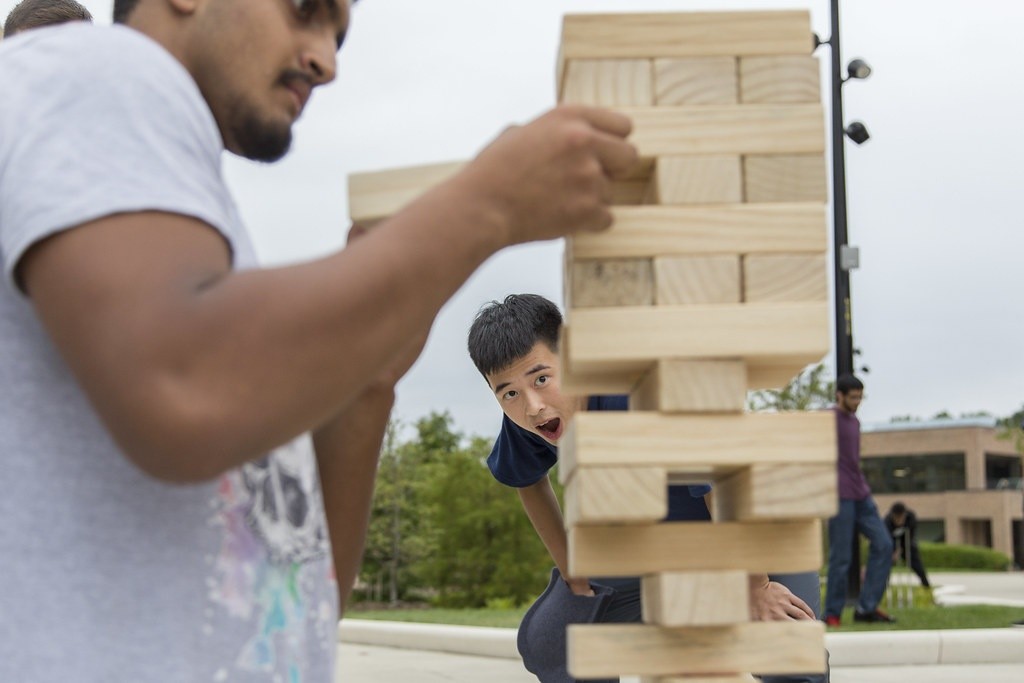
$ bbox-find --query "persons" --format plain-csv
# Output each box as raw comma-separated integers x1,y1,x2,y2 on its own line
0,0,638,683
824,376,897,626
4,0,92,39
468,294,831,683
885,502,929,587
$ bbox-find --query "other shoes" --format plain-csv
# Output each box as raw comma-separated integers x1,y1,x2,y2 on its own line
854,609,895,623
824,614,841,627
